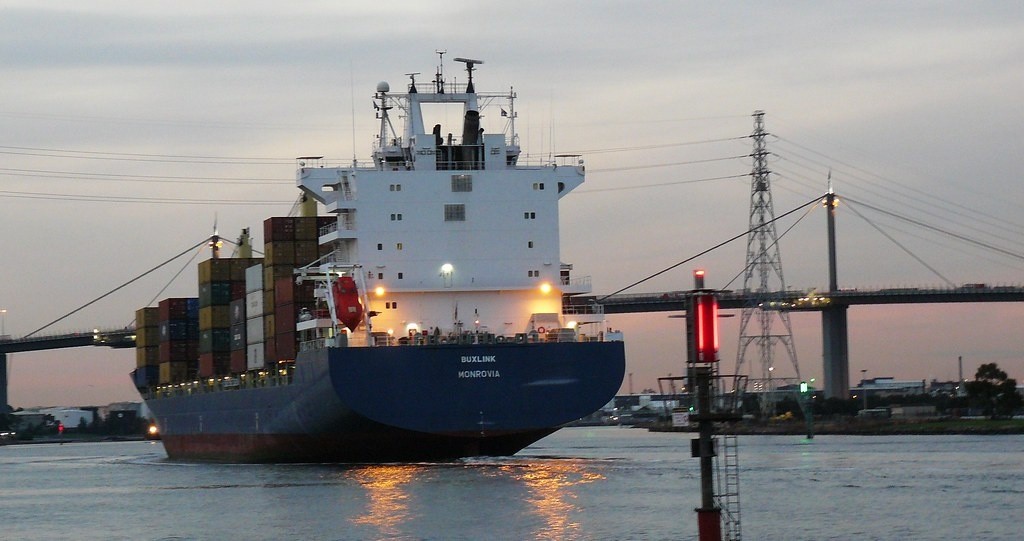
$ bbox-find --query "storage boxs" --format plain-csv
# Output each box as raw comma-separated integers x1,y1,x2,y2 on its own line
134,216,338,390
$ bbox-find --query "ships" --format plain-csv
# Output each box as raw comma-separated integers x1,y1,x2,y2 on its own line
128,50,629,468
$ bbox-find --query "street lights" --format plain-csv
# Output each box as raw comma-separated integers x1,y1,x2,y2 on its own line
0,309,7,335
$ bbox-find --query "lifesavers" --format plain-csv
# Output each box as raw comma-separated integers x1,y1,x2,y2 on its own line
538,326,546,334
